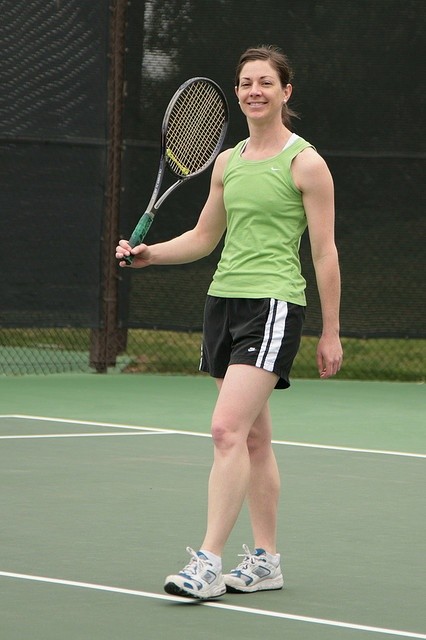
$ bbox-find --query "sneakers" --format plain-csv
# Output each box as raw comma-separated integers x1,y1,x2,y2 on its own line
164,547,226,599
222,544,284,593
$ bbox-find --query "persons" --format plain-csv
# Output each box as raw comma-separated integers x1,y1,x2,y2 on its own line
116,48,343,600
